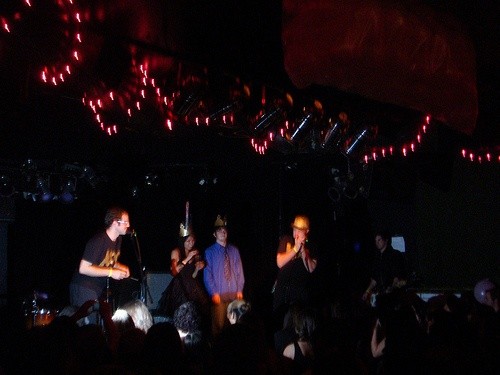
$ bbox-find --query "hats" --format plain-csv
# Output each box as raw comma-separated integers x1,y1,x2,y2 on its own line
292,216,309,231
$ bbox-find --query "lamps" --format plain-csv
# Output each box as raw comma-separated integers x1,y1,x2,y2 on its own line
340,126,374,159
206,95,245,121
319,120,349,153
174,92,198,116
286,112,323,146
250,104,287,138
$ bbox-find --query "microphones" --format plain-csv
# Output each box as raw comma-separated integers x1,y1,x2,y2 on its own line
127,228,136,236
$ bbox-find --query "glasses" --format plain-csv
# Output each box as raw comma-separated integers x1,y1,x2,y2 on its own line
116,221,129,226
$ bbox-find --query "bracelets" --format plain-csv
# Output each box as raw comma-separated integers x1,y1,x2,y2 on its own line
181,262,184,265
108,267,113,277
293,248,298,253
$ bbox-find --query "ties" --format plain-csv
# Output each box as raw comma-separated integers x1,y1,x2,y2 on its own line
223,248,231,281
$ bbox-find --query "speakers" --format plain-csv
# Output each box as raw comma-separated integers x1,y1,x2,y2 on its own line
145,271,174,312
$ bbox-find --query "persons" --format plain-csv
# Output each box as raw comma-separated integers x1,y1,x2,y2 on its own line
25,286,500,375
69,208,130,324
363,233,407,300
151,224,205,321
273,216,319,328
202,215,245,332
